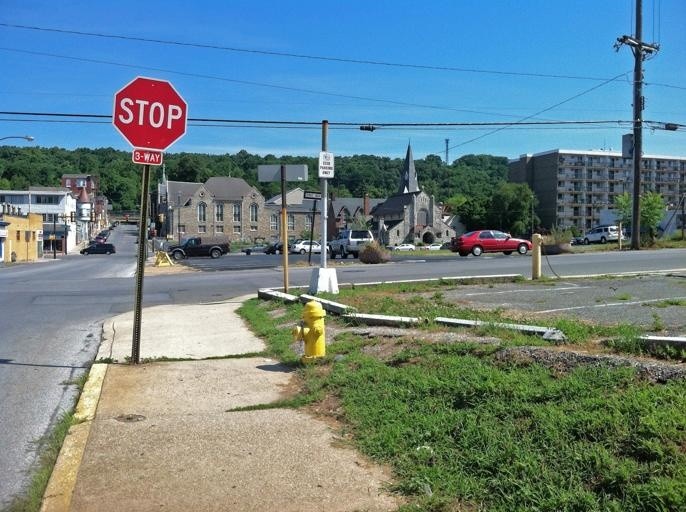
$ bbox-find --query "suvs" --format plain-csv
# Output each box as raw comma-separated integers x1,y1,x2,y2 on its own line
329,229,374,259
582,226,625,245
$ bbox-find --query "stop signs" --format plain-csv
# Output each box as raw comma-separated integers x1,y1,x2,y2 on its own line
110,74,187,166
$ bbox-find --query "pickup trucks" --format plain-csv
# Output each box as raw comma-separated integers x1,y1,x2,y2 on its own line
167,236,229,260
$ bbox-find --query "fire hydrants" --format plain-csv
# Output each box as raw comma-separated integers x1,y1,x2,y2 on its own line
293,297,330,367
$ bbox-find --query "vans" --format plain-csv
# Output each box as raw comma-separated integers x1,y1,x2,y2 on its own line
79,244,116,256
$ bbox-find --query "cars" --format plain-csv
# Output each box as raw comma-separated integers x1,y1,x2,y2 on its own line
395,244,415,251
95,220,119,245
450,229,533,257
291,239,329,254
240,244,268,255
421,244,443,251
